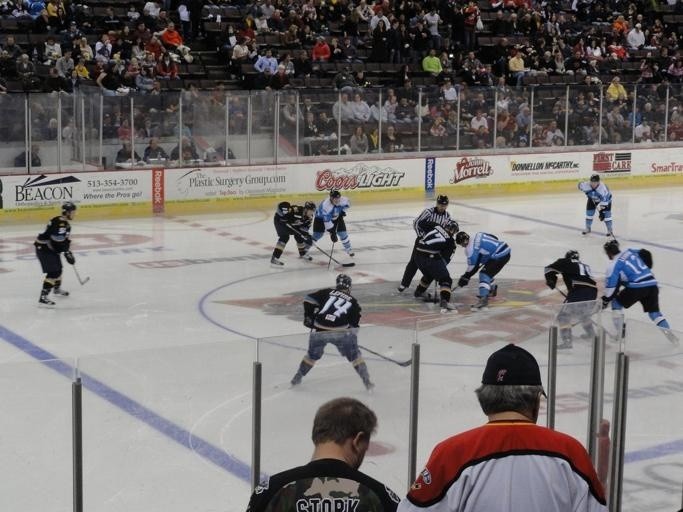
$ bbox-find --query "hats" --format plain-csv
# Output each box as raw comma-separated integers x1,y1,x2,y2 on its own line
482,344,542,386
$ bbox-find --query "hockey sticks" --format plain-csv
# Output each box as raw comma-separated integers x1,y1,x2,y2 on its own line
286,223,355,267
434,279,439,305
555,285,625,342
357,345,412,366
72,264,90,286
327,210,342,271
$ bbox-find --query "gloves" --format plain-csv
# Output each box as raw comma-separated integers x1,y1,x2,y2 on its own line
337,210,346,220
599,208,605,221
458,274,469,287
602,295,608,310
302,231,317,246
547,277,558,289
65,251,75,265
331,233,337,241
279,218,289,224
304,314,315,328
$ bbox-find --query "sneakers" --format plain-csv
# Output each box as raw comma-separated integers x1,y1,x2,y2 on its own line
54,288,69,295
291,374,300,384
471,284,497,307
582,226,591,234
581,329,595,338
300,254,313,261
348,251,354,256
363,380,373,388
271,256,284,264
607,229,612,236
414,291,431,297
398,284,405,292
39,295,55,304
556,338,572,348
440,301,456,309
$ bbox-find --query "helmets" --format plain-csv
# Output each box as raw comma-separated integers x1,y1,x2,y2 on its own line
305,200,316,210
604,241,620,259
337,274,351,290
330,190,340,197
590,174,599,182
565,251,579,261
445,219,469,244
62,201,75,215
437,195,449,204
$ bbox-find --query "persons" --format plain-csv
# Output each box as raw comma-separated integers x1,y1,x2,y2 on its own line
545,249,598,350
246,396,401,512
312,190,355,257
396,193,449,292
601,239,679,343
0,0,683,169
578,173,613,236
270,200,317,265
290,274,375,391
456,231,511,309
414,219,459,309
33,201,76,305
395,344,607,512
